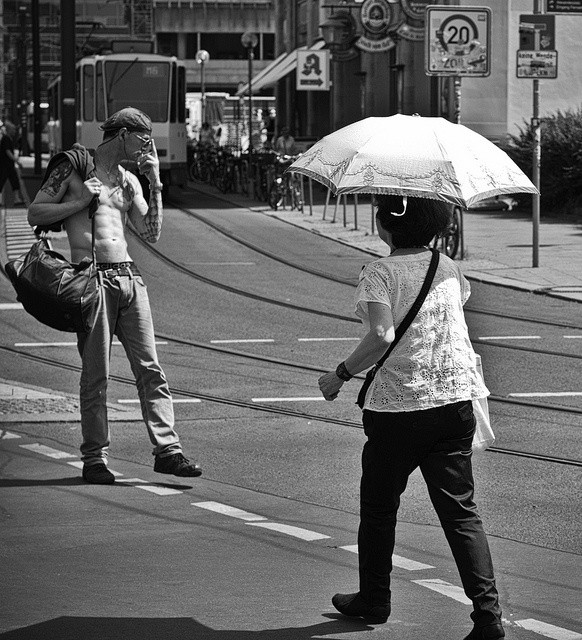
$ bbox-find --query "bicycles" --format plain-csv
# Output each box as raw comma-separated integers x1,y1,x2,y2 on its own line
432,220,459,259
191,140,304,212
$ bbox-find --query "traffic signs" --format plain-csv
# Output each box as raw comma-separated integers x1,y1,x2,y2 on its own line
426,8,490,76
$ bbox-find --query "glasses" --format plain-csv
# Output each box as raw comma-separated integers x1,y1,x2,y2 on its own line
128,132,151,150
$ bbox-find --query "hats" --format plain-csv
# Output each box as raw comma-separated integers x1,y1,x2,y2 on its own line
101,107,152,132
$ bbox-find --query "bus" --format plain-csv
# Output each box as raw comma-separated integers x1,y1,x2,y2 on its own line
47,53,189,171
27,102,47,152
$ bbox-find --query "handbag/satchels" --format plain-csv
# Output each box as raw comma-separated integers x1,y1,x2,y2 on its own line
5,238,98,335
471,354,495,453
357,367,379,409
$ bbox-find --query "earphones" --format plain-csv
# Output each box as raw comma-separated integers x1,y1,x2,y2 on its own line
121,133,125,139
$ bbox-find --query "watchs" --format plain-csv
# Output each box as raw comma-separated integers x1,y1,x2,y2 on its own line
335,361,352,382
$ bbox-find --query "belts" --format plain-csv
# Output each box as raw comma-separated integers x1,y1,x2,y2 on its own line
100,268,142,278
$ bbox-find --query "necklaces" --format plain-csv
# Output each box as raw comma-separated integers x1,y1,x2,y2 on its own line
390,245,426,254
95,148,121,186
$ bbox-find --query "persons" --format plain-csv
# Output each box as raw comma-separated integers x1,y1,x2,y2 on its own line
199,122,218,148
1,123,28,206
27,104,203,485
318,193,507,640
274,126,299,158
149,183,163,191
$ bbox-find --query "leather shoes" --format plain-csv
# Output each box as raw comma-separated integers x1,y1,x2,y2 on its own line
82,463,115,484
154,453,203,477
331,590,390,624
464,623,504,638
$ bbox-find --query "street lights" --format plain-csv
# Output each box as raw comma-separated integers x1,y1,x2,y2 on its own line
195,50,211,124
240,33,257,197
